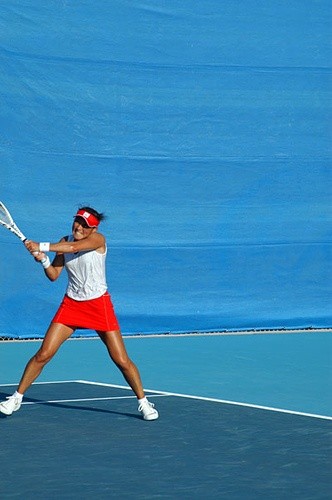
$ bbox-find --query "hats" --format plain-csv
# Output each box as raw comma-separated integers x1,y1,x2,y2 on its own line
72,210,100,228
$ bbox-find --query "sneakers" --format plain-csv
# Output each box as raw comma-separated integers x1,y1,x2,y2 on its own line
137,399,159,420
0,394,22,415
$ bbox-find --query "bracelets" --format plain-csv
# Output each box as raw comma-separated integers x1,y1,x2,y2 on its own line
41,256,50,268
39,242,50,252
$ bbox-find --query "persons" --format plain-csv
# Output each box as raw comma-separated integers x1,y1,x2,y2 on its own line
1,207,159,421
